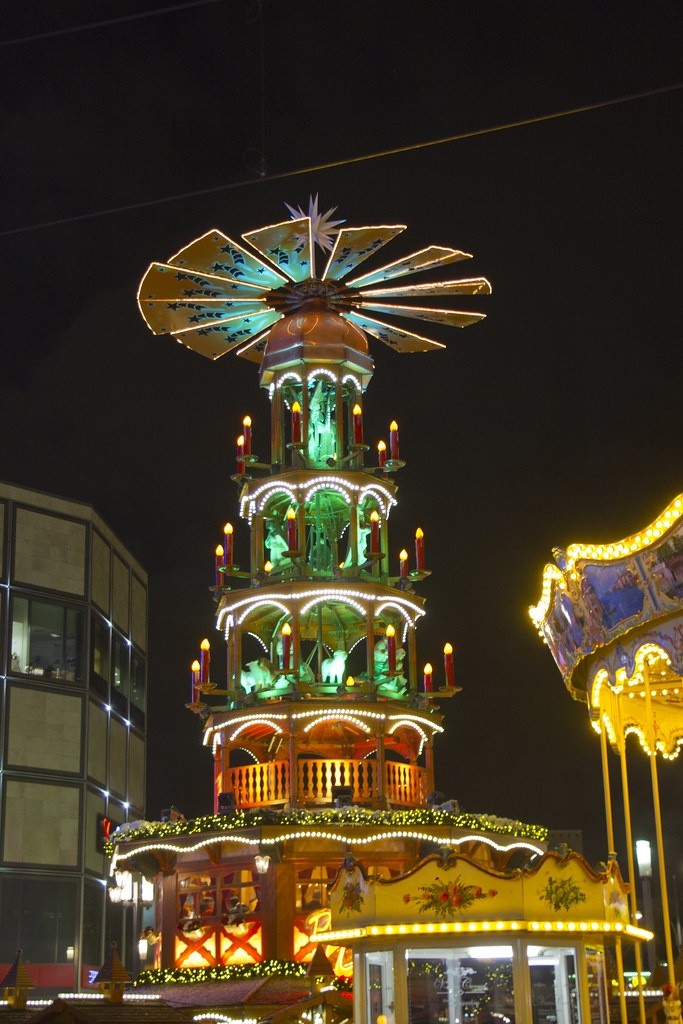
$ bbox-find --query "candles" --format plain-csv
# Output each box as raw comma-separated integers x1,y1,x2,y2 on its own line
370,510,379,552
237,435,245,474
200,638,209,683
423,663,433,704
399,549,408,577
415,527,425,569
191,660,200,703
377,441,389,477
288,507,296,551
443,642,456,688
243,416,252,455
215,545,224,585
223,522,233,565
389,420,400,460
292,401,300,442
386,624,396,671
353,404,363,445
281,622,291,670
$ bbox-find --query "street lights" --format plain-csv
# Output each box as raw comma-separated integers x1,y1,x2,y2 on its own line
108,869,154,984
636,839,656,972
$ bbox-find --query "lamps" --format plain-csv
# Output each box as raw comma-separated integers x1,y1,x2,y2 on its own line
218,792,237,812
427,791,445,810
253,849,272,874
331,785,354,808
108,868,123,903
